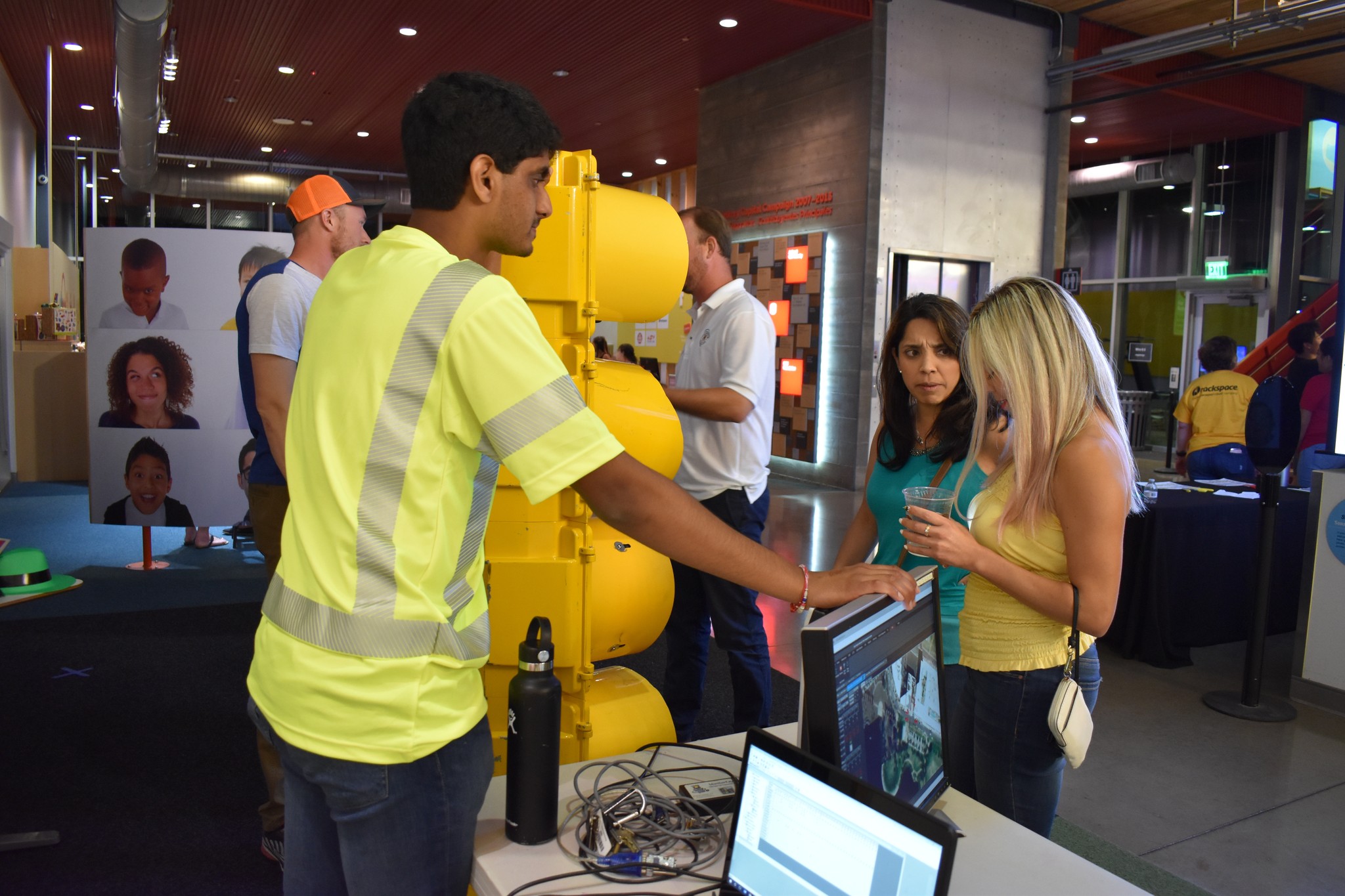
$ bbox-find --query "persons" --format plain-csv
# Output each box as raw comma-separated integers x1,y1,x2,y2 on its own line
222,526,254,536
1173,336,1259,479
591,336,611,360
899,279,1145,837
616,344,638,365
663,207,775,736
103,437,195,527
246,71,919,896
832,292,1014,693
220,246,286,330
98,238,188,329
233,439,256,526
98,336,200,429
184,526,229,548
1285,319,1334,487
236,174,369,871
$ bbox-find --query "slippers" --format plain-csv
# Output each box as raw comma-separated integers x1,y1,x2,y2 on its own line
196,534,229,550
184,537,195,547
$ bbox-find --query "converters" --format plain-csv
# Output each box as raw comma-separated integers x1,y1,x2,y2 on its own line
680,776,740,817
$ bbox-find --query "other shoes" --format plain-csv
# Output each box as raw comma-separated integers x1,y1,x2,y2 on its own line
223,525,254,536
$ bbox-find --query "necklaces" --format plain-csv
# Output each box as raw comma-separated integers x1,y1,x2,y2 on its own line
916,409,928,445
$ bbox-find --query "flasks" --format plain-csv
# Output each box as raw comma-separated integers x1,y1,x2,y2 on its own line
504,616,561,845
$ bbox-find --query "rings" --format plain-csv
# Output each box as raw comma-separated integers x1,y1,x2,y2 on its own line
924,524,931,536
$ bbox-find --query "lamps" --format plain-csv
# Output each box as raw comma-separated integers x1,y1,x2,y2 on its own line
162,46,179,81
158,107,170,135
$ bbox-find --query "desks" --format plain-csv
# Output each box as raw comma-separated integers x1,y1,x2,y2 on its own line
1104,479,1310,669
463,723,1153,896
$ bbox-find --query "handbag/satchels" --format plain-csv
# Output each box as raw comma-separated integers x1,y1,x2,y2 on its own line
1048,675,1095,769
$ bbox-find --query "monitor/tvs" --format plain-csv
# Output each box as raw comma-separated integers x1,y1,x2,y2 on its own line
718,726,958,896
796,565,966,838
1199,345,1247,372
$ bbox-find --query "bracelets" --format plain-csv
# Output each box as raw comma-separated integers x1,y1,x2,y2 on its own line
789,564,809,613
1176,451,1186,456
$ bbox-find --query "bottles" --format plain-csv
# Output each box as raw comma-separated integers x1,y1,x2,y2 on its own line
1143,479,1158,503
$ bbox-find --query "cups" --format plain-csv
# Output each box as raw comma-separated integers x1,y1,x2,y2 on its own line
902,487,958,557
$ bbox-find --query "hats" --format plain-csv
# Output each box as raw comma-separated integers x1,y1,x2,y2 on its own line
285,174,386,227
0,548,76,597
